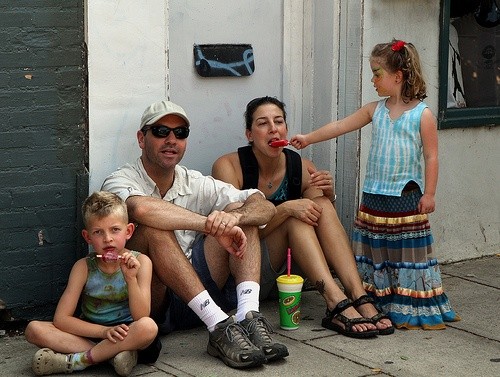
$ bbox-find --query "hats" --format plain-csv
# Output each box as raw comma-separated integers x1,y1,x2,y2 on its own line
139,100,191,134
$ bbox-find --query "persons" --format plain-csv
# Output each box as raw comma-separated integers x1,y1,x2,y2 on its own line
208,97,395,339
99,101,289,366
25,191,163,377
290,39,462,330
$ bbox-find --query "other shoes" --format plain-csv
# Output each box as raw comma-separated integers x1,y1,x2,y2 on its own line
33,348,73,375
112,350,138,376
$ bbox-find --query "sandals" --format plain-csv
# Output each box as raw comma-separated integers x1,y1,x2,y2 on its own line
352,294,395,334
321,299,380,337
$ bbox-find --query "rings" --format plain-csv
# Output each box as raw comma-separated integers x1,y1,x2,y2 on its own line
328,180,329,184
221,223,226,227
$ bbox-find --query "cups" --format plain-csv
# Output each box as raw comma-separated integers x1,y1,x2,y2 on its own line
276,275,304,331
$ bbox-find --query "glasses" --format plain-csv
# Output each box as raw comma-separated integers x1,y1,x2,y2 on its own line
142,124,190,139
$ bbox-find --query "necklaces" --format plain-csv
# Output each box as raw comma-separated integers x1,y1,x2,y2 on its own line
259,156,280,189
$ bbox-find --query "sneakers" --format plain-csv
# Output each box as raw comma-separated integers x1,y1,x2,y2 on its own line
236,310,290,363
206,316,268,369
